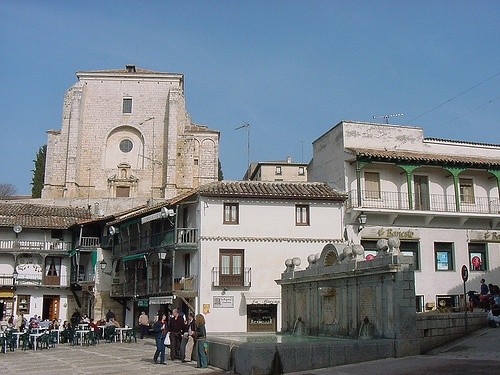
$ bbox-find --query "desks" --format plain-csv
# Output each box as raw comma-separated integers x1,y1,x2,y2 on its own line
12,333,24,348
31,328,48,333
114,328,133,343
29,334,41,351
97,326,105,339
75,331,89,346
51,330,63,344
78,324,88,330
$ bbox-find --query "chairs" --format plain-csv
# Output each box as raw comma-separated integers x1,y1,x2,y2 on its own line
0,319,137,354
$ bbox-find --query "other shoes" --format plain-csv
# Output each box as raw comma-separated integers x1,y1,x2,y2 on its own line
153,359,157,364
182,360,191,363
159,361,166,364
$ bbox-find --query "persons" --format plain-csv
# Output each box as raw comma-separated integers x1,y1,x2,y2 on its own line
480,278,500,328
139,312,149,339
169,304,187,361
189,314,208,368
0,308,121,347
153,310,168,365
182,313,197,363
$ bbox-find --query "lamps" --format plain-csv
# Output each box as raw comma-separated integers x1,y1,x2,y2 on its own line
157,245,171,260
108,226,119,235
356,213,367,233
161,208,175,218
100,259,111,275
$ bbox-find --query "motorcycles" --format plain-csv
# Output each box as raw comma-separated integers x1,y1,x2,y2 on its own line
466,291,495,312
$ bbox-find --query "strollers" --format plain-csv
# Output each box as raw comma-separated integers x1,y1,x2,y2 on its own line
487,297,500,326
